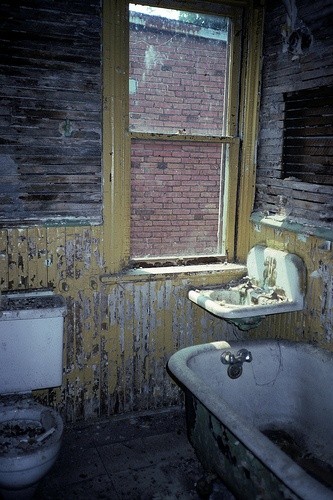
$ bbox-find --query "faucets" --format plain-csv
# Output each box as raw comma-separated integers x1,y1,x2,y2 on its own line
220,349,253,379
239,276,255,288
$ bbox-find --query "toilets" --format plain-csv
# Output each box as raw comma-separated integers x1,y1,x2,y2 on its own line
0,288,69,500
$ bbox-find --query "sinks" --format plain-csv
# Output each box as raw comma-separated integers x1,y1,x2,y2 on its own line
188,244,308,331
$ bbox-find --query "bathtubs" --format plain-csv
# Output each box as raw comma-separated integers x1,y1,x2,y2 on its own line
167,336,332,500
